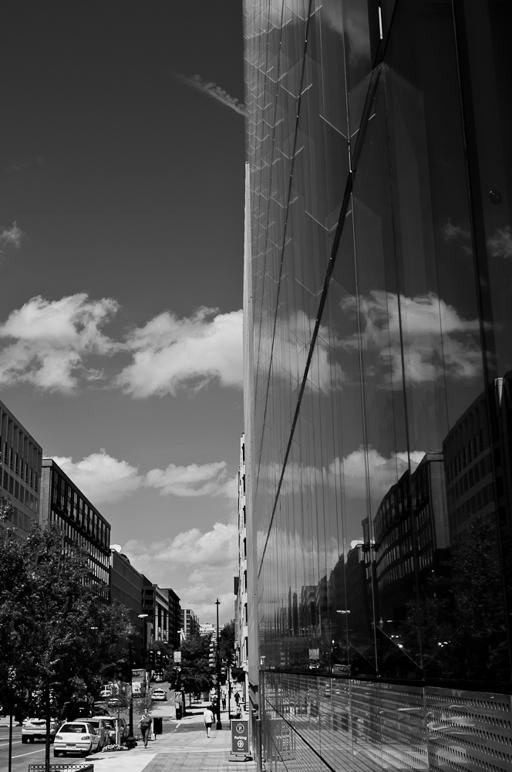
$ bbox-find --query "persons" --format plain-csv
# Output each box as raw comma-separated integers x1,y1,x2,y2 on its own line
204,702,215,738
222,691,226,710
234,691,240,706
140,709,152,748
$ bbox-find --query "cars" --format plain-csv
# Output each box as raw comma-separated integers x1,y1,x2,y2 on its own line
21,672,169,755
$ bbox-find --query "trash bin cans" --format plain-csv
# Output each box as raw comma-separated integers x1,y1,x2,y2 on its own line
176,708,182,720
153,717,162,734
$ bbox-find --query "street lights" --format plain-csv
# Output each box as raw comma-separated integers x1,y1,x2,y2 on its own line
215,597,224,730
136,613,193,709
